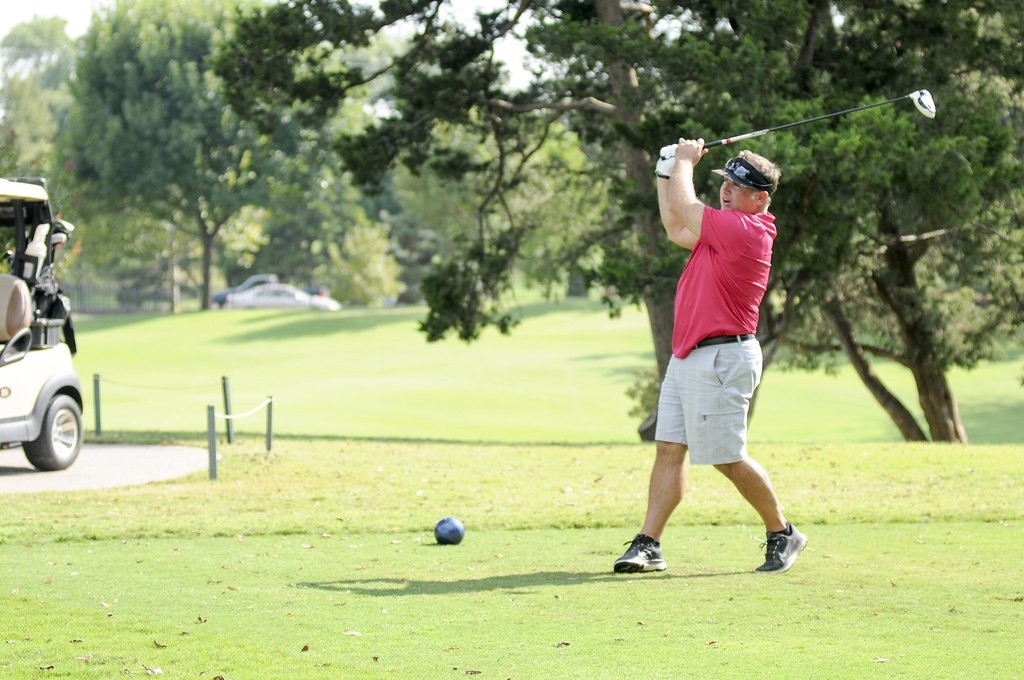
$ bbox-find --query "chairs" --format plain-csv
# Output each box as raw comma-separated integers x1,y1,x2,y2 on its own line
0,273,33,355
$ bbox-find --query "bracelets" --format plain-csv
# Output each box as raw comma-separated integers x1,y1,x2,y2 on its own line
656,171,673,181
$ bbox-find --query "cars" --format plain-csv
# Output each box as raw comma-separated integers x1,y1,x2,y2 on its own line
226,284,342,311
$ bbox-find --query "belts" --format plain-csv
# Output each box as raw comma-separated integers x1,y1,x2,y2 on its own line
692,332,756,350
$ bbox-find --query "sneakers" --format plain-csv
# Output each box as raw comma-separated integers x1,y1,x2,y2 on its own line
613,533,668,573
754,522,808,573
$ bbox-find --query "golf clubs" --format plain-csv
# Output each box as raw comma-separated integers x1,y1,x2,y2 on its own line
658,89,936,160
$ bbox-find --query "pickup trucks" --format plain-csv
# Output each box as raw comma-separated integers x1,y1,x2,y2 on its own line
211,274,328,309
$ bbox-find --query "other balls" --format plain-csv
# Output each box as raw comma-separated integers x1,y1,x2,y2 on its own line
435,517,464,544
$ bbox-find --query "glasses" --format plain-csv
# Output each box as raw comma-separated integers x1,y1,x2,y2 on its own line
724,157,772,188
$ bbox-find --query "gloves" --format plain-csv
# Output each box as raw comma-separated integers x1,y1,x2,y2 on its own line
654,144,678,177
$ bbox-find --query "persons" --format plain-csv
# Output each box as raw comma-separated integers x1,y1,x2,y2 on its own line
613,135,809,576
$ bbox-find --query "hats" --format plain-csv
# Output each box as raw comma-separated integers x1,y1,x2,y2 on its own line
711,157,773,197
